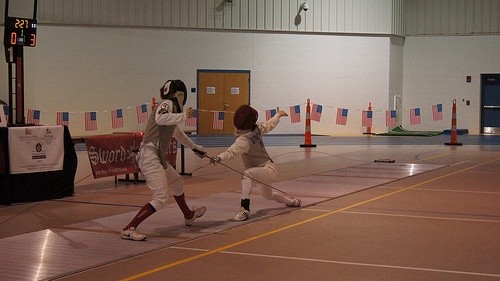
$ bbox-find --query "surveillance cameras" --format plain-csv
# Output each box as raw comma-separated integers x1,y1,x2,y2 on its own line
302,4,308,11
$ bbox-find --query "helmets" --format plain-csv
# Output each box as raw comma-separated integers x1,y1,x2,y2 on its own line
161,80,187,106
234,106,259,130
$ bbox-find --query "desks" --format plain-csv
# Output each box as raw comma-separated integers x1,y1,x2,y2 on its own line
71,130,192,185
0,124,78,204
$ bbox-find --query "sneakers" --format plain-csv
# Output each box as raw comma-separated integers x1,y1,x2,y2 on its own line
233,207,252,221
120,229,147,241
288,197,301,207
186,207,207,226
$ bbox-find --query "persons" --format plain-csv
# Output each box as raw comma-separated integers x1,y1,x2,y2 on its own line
216,104,301,222
121,80,208,241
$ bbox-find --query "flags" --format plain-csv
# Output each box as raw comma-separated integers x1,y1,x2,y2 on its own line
111,109,123,129
185,109,197,126
386,111,396,126
432,104,442,121
27,109,40,125
213,112,224,129
410,108,421,125
311,104,322,121
57,112,69,126
266,110,276,121
136,105,148,123
85,112,97,131
336,108,348,126
290,106,300,123
362,111,372,127
